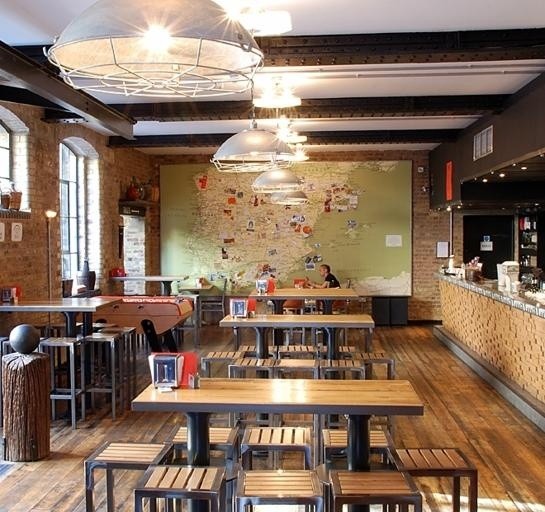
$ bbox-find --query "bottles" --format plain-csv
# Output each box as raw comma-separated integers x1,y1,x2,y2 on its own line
449,253,455,272
188,371,201,390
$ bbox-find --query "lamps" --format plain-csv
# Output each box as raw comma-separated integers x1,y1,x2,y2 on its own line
42,1,292,98
210,83,306,206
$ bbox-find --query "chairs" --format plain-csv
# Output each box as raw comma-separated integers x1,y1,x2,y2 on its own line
178,276,353,345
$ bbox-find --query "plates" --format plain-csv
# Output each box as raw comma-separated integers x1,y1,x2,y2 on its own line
445,267,459,275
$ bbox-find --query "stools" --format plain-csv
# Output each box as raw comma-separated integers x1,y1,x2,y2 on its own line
355,350,395,435
0,323,138,431
203,343,366,384
84,424,481,511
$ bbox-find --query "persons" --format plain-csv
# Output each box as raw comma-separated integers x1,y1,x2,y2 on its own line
307,264,340,288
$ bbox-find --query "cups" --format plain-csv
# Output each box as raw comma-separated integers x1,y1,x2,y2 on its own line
247,311,256,318
